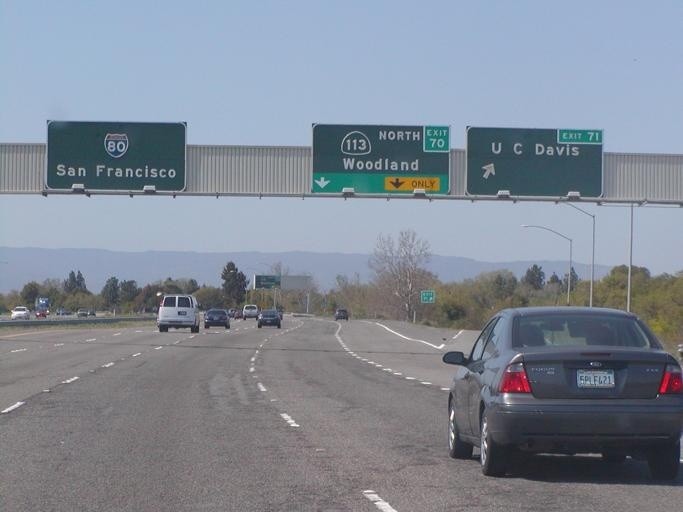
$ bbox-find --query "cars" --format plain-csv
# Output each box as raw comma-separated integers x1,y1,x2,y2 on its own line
257,310,281,328
203,308,231,329
442,305,683,480
11,306,96,320
156,295,200,334
334,308,349,321
228,304,258,320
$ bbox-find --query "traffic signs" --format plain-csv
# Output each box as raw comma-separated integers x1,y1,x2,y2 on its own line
463,123,605,198
43,119,189,193
310,122,451,195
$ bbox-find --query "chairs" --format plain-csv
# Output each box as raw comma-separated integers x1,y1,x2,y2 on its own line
519,325,546,348
583,326,617,349
